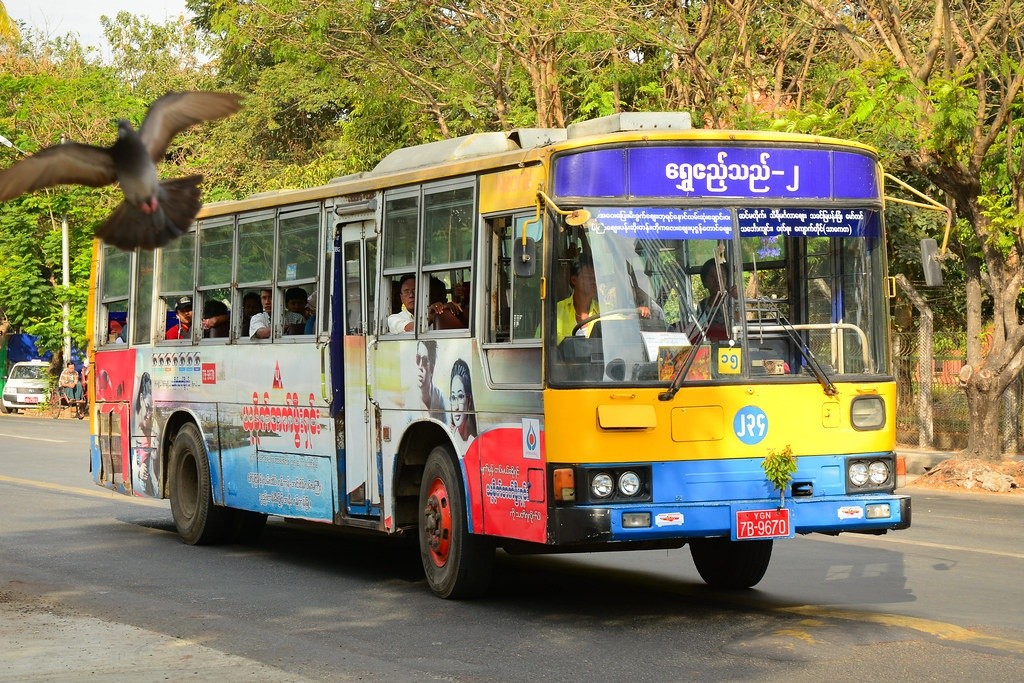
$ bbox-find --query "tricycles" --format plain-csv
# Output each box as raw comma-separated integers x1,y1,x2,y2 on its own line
49,386,90,420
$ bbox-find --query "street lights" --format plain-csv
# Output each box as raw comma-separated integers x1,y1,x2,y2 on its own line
0,134,74,369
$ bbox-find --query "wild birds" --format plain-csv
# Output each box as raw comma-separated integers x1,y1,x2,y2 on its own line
1,90,244,252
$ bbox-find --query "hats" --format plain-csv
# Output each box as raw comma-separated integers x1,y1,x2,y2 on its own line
174,297,192,313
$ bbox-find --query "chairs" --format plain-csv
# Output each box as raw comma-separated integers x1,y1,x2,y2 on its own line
210,304,469,338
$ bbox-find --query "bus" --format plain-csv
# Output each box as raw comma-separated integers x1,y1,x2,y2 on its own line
86,112,953,601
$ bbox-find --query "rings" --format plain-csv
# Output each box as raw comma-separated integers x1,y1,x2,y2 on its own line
435,305,438,307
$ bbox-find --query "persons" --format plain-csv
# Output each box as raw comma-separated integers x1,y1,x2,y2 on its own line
535,257,651,345
450,358,477,457
61,362,82,401
79,352,88,409
682,258,738,345
133,372,167,497
115,249,472,344
401,340,446,426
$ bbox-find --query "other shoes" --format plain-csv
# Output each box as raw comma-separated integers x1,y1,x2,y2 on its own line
69,398,75,405
77,401,82,404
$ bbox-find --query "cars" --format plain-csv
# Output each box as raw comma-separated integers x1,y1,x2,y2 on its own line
0,359,53,414
